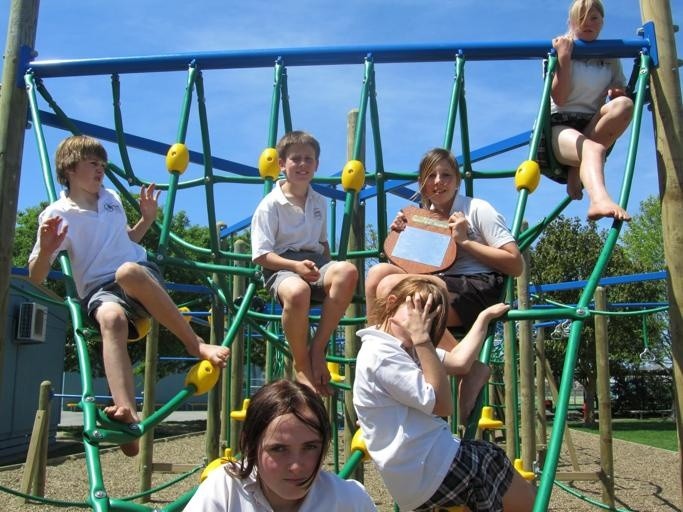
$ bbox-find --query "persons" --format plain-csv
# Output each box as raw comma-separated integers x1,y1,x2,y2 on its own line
250,131,360,397
182,378,377,512
363,147,525,423
27,135,231,456
351,275,537,511
529,1,635,221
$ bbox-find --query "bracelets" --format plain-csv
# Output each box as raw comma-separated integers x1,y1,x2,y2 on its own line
413,339,433,347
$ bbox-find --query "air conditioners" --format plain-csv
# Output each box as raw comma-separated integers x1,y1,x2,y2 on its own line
17,302,48,343
